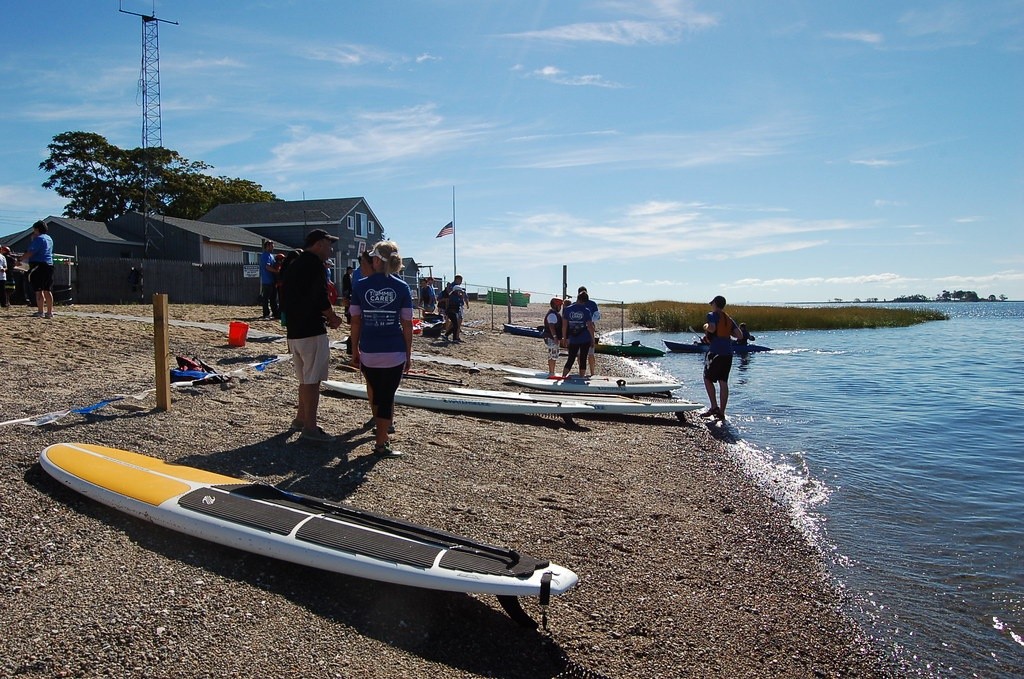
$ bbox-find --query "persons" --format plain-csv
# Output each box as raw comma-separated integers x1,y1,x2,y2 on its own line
0,247,16,306
342,243,413,458
562,286,600,375
738,324,751,346
418,277,436,314
544,298,564,373
437,275,469,343
700,296,743,419
19,221,54,317
261,233,342,441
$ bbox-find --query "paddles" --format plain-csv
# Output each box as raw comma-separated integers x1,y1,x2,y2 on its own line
688,325,700,338
398,387,563,405
239,468,522,564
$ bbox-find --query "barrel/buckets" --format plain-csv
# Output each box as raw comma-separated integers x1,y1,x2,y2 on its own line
228,321,250,347
413,319,421,334
423,312,443,337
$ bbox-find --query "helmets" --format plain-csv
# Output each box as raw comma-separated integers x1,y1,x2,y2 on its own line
2,246,10,255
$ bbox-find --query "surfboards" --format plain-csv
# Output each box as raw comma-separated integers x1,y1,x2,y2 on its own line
449,385,706,414
36,439,581,597
502,376,684,395
499,366,664,384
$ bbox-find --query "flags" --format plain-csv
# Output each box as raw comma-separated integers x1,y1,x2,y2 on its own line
436,221,453,238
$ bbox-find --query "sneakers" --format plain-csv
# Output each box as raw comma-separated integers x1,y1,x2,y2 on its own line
44,312,54,319
291,418,303,430
441,334,449,342
452,338,463,344
32,312,46,318
303,426,336,443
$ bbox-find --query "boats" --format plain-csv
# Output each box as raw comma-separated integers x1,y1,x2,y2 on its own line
503,321,550,341
663,334,775,357
589,339,665,360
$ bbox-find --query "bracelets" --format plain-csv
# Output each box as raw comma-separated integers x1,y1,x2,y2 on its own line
553,335,557,337
562,337,566,339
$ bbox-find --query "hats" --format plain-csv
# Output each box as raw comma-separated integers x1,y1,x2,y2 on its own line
326,259,335,265
709,295,726,309
368,247,388,262
305,228,339,245
346,267,354,269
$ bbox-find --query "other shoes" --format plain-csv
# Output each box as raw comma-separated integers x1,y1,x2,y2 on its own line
363,417,376,429
372,424,396,435
714,414,727,421
700,406,721,417
375,440,404,459
